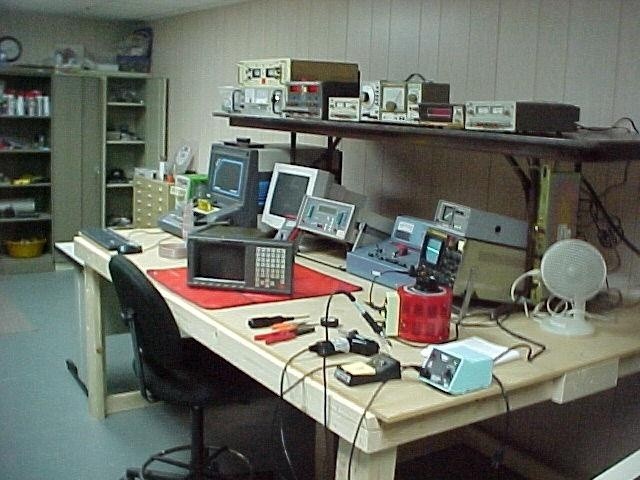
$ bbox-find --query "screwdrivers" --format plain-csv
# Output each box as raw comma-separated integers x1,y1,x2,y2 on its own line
248,314,310,329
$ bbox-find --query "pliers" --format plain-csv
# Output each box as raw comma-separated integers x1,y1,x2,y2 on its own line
272,321,305,330
253,326,317,346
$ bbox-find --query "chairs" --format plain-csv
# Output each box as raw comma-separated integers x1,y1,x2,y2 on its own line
109,252,280,479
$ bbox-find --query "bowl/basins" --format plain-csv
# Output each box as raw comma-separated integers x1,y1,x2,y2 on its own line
5,240,47,257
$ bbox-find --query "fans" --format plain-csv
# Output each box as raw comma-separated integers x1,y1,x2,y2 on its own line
534,234,612,341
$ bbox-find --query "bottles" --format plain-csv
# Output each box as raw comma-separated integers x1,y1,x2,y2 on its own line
183,205,193,242
53,47,84,72
0,94,50,116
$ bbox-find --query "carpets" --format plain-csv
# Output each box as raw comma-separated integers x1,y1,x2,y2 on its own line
0,285,40,338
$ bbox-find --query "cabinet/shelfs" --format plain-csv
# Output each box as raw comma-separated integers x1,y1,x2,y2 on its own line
126,172,189,229
98,68,170,233
0,65,58,274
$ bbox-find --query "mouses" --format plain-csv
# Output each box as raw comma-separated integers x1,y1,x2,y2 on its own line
118,243,141,254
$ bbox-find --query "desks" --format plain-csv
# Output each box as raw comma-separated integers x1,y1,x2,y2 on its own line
46,227,139,398
71,109,640,480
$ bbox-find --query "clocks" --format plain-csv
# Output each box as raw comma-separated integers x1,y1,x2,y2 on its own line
1,34,24,62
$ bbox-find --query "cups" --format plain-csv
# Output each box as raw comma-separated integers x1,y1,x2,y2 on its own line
145,160,175,182
106,95,143,226
0,198,35,217
159,243,187,259
197,198,211,211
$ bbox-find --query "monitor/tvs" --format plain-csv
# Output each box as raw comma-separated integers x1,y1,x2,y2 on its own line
187,225,268,291
261,162,368,239
207,142,258,207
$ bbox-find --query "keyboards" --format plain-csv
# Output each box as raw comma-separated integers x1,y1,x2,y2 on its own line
81,228,134,250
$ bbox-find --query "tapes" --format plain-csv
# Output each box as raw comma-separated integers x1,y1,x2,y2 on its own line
321,316,338,327
